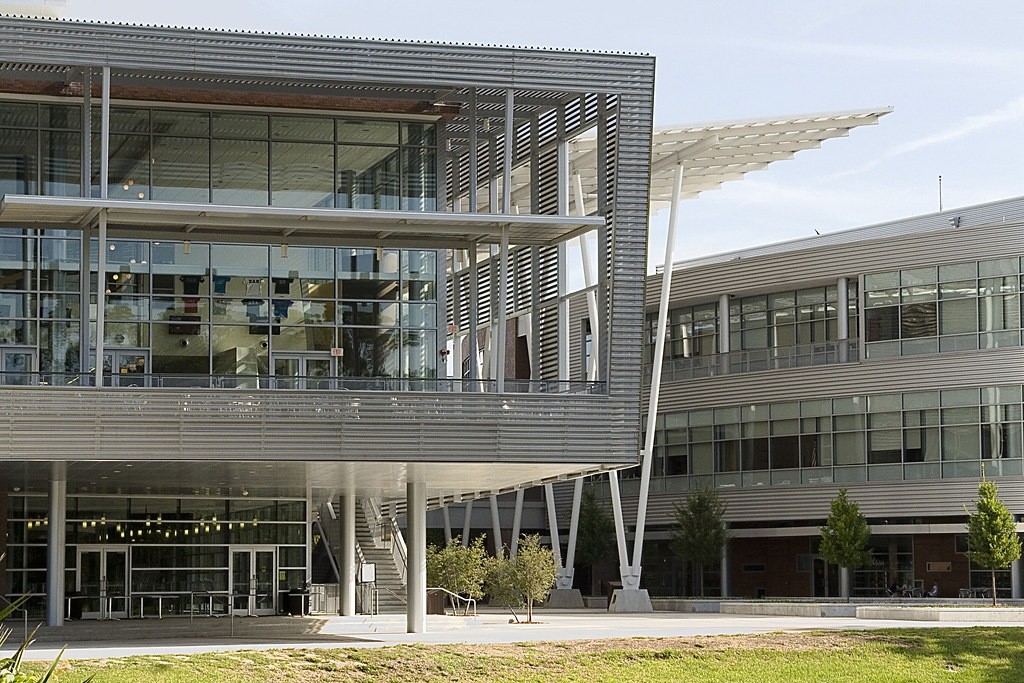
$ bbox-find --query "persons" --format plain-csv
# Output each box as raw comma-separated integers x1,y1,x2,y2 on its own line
902,578,913,598
927,580,939,597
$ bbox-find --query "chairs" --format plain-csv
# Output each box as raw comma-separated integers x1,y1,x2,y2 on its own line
884,582,996,600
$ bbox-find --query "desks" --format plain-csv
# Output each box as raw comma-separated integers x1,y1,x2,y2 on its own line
968,587,988,598
63,591,324,618
